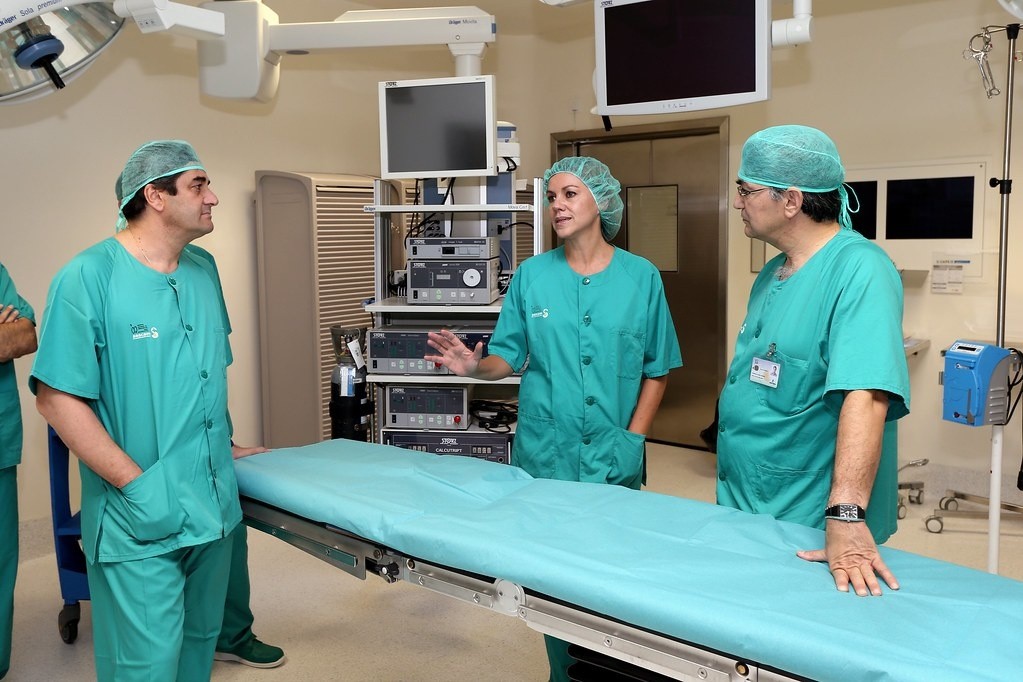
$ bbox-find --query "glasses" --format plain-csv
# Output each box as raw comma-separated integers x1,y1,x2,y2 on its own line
737,185,776,196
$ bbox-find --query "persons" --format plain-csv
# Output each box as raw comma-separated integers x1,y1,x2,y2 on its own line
423,158,683,681
0,260,37,679
30,141,272,682
716,124,910,595
201,393,284,668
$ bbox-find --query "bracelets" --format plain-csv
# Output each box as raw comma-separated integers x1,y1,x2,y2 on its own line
229,437,235,448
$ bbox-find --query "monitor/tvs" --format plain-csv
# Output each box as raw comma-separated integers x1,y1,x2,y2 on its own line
840,164,984,271
590,0,772,115
377,74,499,180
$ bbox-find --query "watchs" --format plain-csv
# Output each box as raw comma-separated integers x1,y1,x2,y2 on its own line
821,500,867,523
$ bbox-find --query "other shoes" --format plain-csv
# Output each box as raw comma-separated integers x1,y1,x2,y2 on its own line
214,633,284,669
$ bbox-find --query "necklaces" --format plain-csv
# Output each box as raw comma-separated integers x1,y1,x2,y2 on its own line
126,226,152,268
